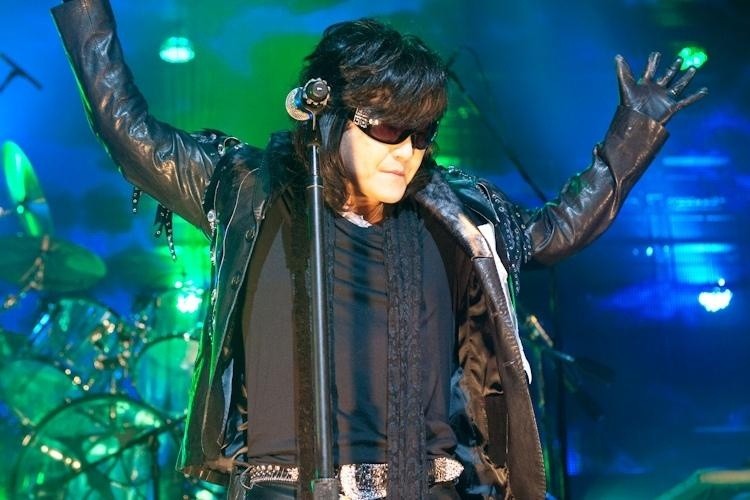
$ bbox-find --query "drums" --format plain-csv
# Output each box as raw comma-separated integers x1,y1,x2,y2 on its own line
5,394,186,500
3,296,138,429
126,281,204,422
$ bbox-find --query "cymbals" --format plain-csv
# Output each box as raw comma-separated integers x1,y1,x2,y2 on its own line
0,235,108,297
111,240,207,290
0,136,55,238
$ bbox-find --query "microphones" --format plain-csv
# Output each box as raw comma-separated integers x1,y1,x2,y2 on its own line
285,77,330,121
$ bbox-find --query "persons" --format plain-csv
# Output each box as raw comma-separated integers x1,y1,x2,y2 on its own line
47,0,710,500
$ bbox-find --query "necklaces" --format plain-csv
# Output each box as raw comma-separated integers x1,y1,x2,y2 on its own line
345,201,385,228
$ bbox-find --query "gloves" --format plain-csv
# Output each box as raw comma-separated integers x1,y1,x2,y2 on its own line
614,51,709,125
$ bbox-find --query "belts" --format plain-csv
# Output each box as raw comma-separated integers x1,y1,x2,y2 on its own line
250,457,464,500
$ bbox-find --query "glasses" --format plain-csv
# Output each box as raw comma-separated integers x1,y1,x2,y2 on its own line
332,103,439,150
